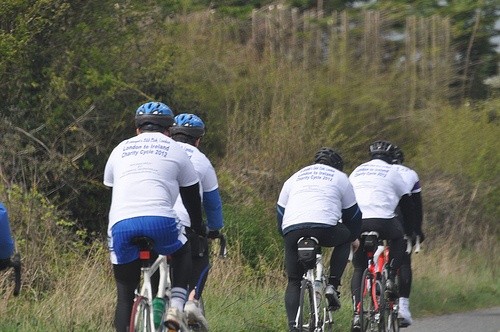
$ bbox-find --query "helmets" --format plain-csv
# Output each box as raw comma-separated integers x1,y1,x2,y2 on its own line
313,147,344,171
169,113,206,140
134,101,175,129
367,140,404,165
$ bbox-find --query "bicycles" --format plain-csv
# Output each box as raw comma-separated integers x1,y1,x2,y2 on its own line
292,237,354,332
129,228,227,332
358,231,421,332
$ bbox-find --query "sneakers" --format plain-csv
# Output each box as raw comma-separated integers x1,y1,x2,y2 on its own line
396,306,413,327
163,307,190,332
184,299,209,332
324,286,342,308
350,316,362,332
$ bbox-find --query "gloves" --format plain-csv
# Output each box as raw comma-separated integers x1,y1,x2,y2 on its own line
208,230,220,240
407,230,417,248
195,223,210,239
417,231,425,243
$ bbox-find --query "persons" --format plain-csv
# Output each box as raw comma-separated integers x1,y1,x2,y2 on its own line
0,203,15,271
277,147,362,332
348,140,425,332
104,102,223,332
